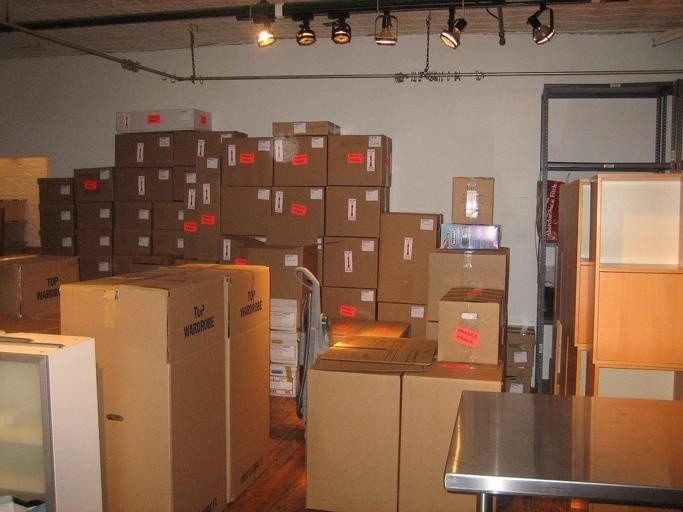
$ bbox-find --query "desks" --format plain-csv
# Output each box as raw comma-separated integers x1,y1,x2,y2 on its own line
443,390,683,512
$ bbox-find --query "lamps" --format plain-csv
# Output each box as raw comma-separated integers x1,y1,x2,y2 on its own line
526,1,555,45
250,0,399,48
440,5,467,49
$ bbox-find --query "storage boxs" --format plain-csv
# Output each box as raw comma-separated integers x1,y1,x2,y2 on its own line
398,339,506,512
272,120,341,137
59,267,227,512
115,108,212,134
0,131,511,397
167,262,270,505
305,336,420,512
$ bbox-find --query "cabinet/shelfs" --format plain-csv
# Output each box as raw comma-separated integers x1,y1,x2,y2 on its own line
535,79,683,400
0,331,103,511
